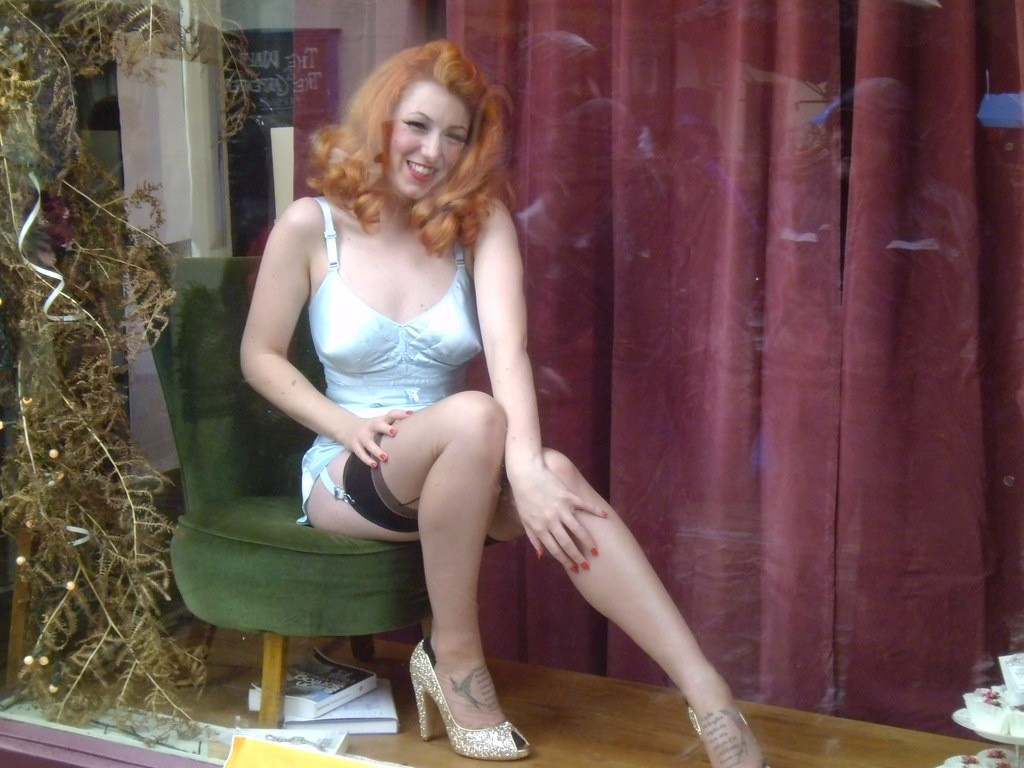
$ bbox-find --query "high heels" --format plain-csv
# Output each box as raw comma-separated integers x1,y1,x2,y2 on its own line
408,638,532,761
688,705,771,768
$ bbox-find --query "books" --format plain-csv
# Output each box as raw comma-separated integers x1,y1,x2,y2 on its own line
240,728,351,756
248,647,378,719
283,678,400,734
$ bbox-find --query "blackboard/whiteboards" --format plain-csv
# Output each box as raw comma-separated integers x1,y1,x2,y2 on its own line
222,28,341,257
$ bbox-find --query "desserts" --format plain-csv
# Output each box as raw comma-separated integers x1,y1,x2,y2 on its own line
940,747,1022,768
962,684,1024,739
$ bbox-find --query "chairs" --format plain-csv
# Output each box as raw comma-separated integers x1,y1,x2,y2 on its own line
126,240,455,728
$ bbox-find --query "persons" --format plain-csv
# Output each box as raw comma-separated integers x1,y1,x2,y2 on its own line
237,39,769,768
